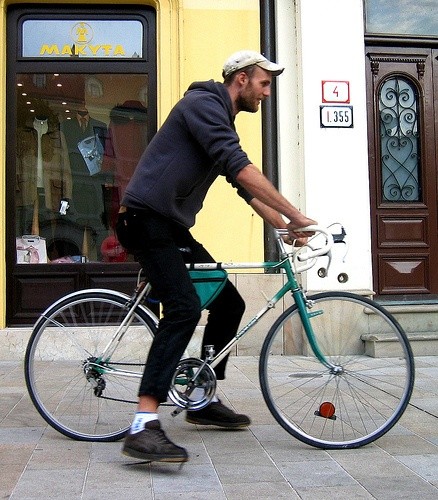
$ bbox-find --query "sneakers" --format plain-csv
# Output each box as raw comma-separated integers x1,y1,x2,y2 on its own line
187,398,251,428
121,418,189,462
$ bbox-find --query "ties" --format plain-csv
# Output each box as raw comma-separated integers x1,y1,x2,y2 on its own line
80,118,87,134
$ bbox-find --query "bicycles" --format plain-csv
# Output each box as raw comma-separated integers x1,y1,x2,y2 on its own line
23,224,415,448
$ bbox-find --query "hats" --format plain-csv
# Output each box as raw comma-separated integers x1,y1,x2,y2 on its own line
221,49,284,78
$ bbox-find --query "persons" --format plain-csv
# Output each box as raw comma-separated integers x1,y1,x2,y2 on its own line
114,50,316,465
